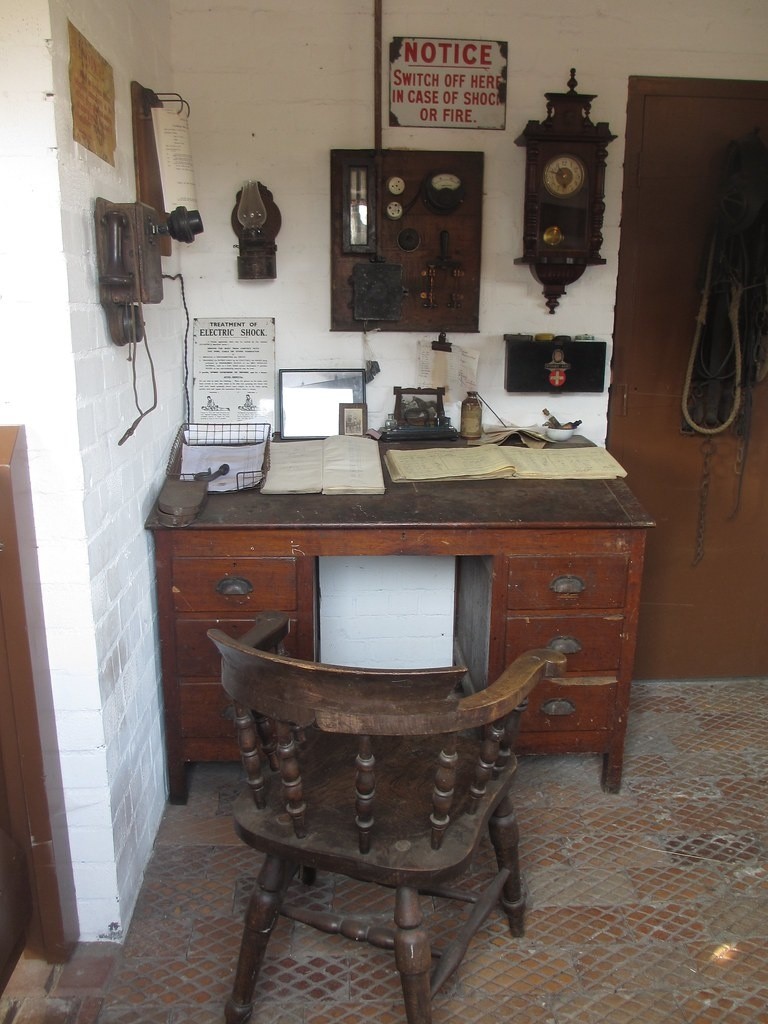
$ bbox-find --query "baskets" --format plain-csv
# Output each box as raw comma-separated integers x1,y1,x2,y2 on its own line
165,422,273,495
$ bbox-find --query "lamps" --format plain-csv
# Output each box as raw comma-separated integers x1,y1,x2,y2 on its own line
231,180,282,279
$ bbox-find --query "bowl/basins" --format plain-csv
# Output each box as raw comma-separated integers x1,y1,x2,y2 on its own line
547,424,574,442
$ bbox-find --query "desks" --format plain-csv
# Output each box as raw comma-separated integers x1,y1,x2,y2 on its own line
144,435,658,803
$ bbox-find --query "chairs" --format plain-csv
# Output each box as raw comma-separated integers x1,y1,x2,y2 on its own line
208,610,569,1024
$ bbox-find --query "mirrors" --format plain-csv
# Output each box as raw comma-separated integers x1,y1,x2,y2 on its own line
279,369,366,440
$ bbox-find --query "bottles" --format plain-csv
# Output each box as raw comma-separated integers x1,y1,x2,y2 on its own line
460,391,483,440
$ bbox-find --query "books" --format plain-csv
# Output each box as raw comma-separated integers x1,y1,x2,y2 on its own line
259,435,385,494
384,444,627,483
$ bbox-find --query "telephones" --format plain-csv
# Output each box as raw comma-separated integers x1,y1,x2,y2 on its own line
94,197,165,348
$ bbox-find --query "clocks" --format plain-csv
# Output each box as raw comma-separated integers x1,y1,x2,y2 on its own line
513,67,617,314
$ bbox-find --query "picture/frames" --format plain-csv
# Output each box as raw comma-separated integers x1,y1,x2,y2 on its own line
339,403,369,437
